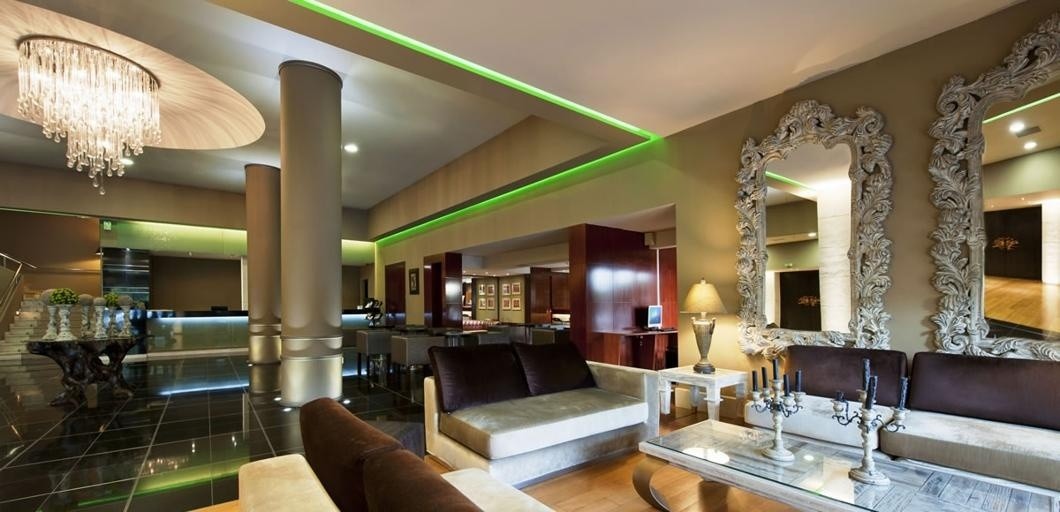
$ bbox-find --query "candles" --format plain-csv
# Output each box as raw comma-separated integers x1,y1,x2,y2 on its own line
746,360,808,398
831,356,911,416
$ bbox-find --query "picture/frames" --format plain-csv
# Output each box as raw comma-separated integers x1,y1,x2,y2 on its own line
476,281,523,310
407,265,421,295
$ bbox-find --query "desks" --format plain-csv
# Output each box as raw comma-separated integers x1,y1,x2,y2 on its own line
597,328,678,370
22,334,154,411
355,321,561,406
655,361,750,426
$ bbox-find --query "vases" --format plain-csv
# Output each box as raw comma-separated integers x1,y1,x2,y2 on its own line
40,304,139,342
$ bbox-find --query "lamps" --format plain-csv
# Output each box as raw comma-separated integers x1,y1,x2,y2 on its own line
676,277,728,377
12,33,170,201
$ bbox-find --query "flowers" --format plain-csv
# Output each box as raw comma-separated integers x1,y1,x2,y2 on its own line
37,287,146,310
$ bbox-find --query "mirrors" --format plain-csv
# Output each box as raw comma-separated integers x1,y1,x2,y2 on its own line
730,94,903,358
921,8,1059,366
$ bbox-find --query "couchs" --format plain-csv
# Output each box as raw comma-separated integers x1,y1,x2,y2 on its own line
881,351,1058,495
418,344,661,489
738,343,910,459
237,395,561,510
